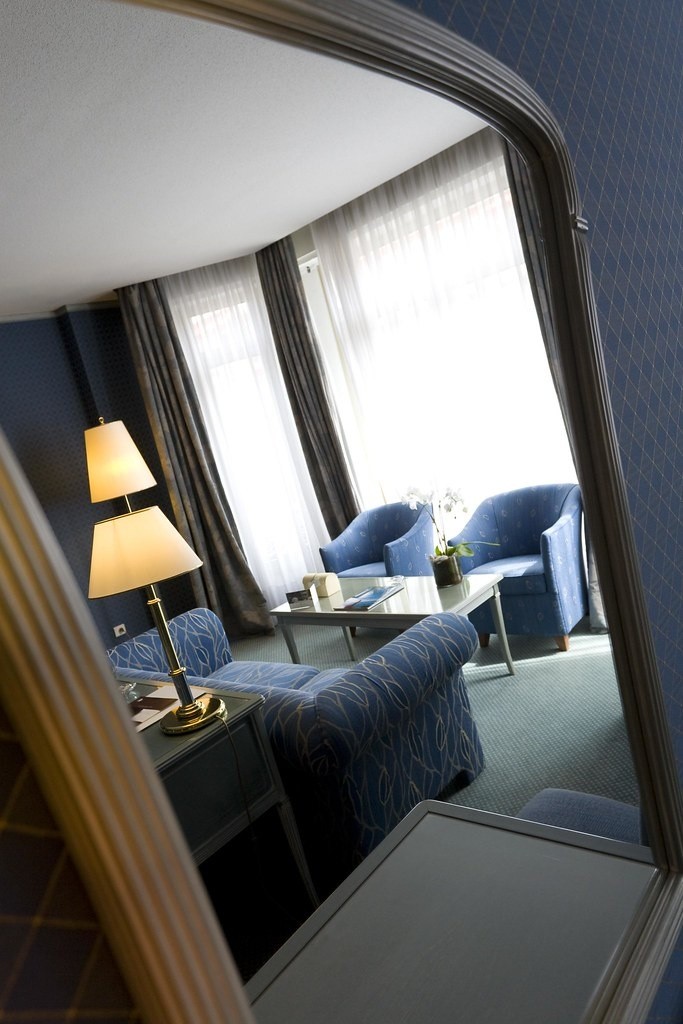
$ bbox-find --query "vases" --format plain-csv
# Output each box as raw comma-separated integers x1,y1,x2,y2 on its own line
431,556,463,588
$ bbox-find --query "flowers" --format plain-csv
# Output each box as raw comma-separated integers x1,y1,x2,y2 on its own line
400,484,500,565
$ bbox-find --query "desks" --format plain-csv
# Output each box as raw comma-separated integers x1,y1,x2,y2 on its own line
242,800,666,1024
116,678,320,908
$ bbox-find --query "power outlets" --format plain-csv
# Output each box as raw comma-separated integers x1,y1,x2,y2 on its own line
113,623,127,637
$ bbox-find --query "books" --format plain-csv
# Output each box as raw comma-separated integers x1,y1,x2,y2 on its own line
333,584,405,611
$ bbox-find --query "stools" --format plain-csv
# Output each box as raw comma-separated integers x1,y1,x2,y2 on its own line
514,787,640,844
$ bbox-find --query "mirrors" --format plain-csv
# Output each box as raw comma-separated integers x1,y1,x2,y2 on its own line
0,0,683,1024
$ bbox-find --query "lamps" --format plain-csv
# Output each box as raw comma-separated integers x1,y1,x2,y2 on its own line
83,417,226,737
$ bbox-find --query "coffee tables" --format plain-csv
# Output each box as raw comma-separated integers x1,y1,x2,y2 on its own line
269,573,515,675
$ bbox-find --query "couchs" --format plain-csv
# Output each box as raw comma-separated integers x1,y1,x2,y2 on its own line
447,483,588,652
319,504,435,638
105,607,486,881
